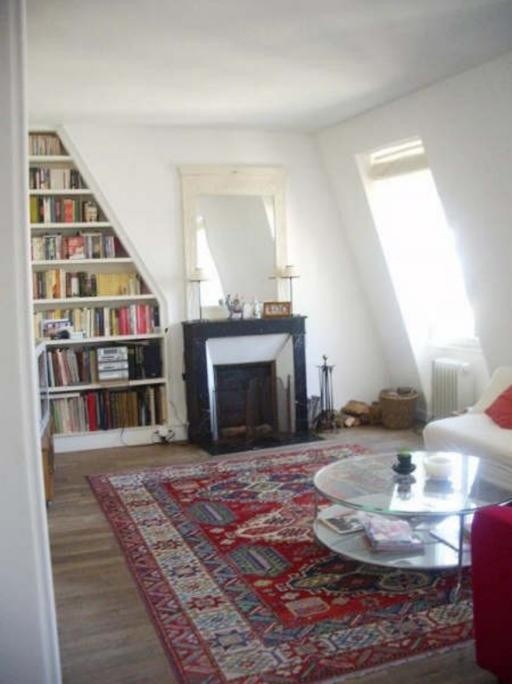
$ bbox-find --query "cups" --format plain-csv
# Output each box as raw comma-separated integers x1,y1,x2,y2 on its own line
397,447,411,466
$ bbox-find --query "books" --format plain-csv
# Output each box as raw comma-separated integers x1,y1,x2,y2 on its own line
29,133,62,155
365,515,422,552
321,510,364,535
30,162,87,189
33,268,150,300
35,340,162,388
34,304,161,339
41,385,166,434
30,195,98,223
31,230,130,261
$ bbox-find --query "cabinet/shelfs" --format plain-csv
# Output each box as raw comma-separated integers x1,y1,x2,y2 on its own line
31,131,172,453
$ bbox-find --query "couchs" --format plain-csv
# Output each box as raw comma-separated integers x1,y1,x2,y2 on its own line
421,365,512,684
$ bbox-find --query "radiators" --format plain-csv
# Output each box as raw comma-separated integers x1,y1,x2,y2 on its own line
430,362,471,419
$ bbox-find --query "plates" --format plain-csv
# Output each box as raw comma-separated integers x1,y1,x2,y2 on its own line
393,462,416,474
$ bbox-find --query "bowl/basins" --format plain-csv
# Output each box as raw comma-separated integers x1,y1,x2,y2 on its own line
424,458,453,482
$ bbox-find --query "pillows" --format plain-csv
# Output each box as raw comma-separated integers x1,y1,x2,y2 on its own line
484,383,512,429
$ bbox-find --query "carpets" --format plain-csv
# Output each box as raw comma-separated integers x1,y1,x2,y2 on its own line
85,442,477,684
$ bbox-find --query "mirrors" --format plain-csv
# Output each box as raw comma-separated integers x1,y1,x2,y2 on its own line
179,161,289,323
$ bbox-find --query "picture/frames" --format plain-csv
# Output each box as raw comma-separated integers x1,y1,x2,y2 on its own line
263,301,292,319
